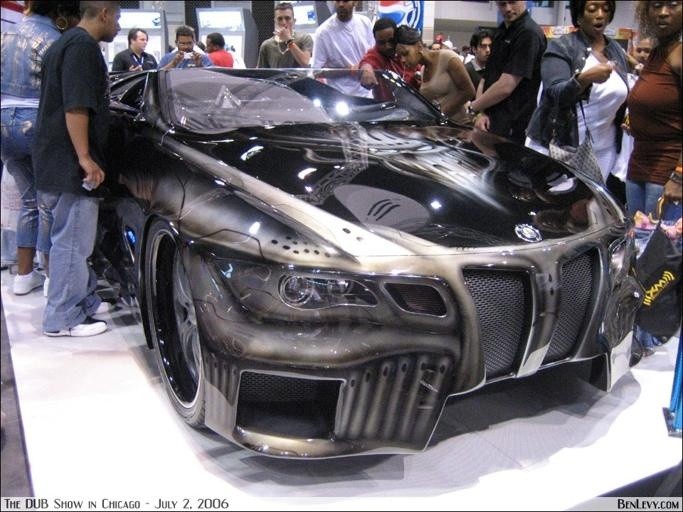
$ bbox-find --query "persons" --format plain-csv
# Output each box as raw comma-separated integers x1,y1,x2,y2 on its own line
32,0,123,338
111,27,158,71
0,0,81,297
157,25,214,69
257,2,314,68
204,33,234,68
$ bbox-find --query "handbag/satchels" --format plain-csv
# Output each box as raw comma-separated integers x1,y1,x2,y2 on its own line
633,194,682,345
546,79,605,188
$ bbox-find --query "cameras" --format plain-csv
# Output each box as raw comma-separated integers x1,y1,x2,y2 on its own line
184,52,193,60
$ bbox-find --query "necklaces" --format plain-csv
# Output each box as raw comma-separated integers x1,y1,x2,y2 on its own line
275,32,294,55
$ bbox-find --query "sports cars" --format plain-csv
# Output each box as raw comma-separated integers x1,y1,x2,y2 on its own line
89,66,645,462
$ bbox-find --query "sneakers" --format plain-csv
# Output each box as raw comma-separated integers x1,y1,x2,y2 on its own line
96,297,117,314
14,269,49,297
44,314,108,338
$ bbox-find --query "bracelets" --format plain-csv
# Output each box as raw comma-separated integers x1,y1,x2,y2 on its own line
286,38,294,47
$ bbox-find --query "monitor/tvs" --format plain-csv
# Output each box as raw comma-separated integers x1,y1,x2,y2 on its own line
199,32,243,56
106,32,163,73
